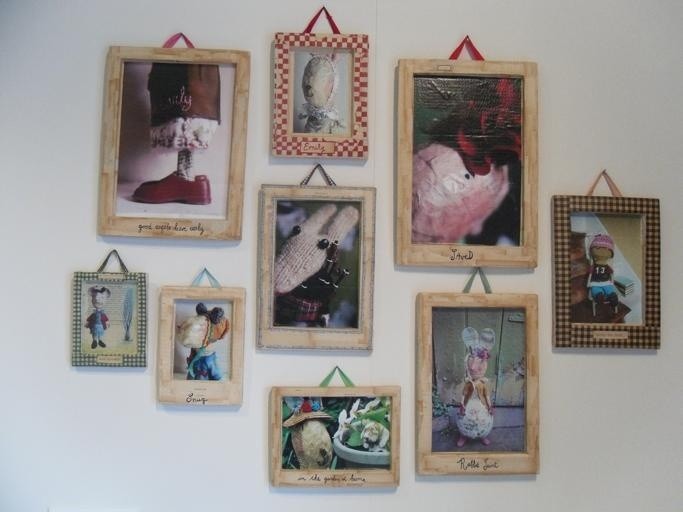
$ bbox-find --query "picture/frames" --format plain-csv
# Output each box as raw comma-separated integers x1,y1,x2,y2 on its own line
551,193,661,350
396,57,539,270
416,292,541,474
270,386,401,487
256,187,376,353
98,45,252,240
71,271,147,368
158,285,247,405
273,32,370,161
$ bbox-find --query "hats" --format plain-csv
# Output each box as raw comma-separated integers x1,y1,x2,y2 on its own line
589,231,617,258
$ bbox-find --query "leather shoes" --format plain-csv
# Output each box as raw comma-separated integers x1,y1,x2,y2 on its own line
131,172,212,206
